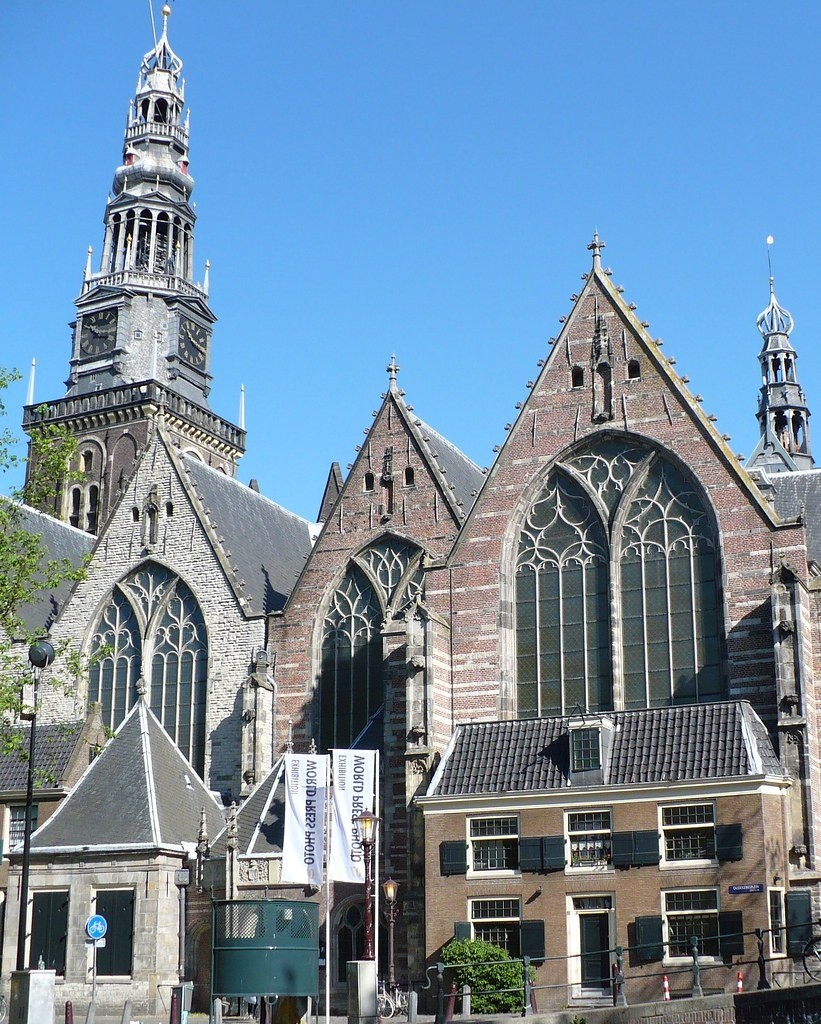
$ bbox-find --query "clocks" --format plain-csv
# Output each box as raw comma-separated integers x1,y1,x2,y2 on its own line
178,315,207,371
80,307,118,358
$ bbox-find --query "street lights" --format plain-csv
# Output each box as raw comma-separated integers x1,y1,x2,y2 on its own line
14,640,56,970
351,805,383,957
379,876,401,1016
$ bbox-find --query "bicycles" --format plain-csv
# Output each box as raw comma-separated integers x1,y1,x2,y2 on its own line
376,980,409,1019
803,918,821,983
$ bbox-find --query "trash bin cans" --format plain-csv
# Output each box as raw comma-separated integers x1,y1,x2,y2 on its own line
345,959,379,1018
8,968,57,1024
169,981,194,1024
